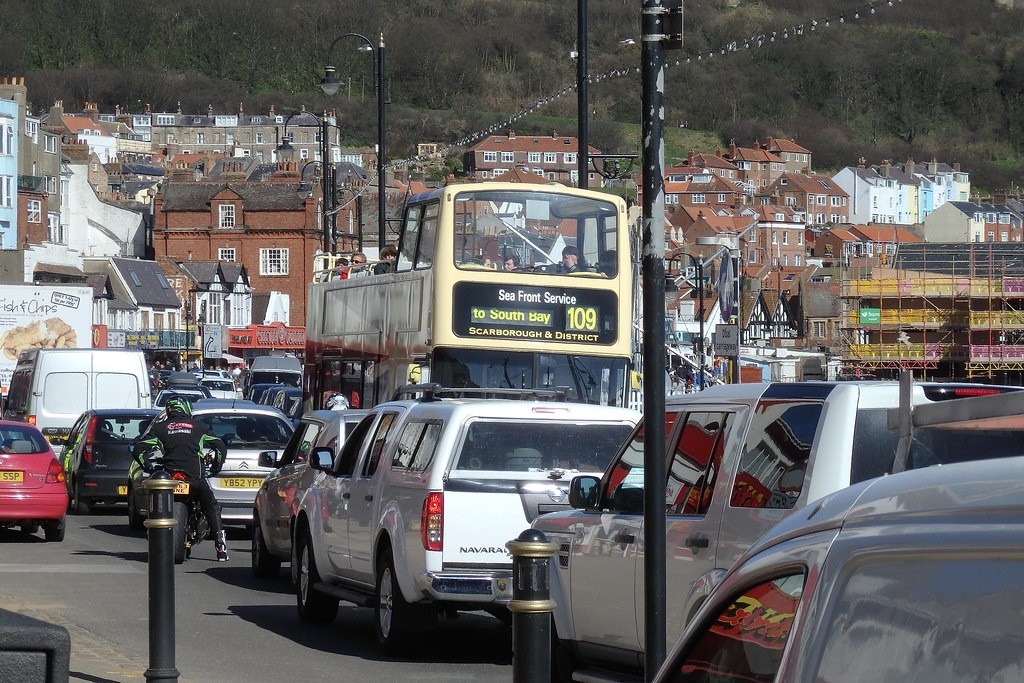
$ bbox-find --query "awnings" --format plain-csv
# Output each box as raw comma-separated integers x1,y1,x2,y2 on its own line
222,353,244,363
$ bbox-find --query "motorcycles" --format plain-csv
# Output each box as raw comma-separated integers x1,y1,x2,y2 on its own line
148,435,233,565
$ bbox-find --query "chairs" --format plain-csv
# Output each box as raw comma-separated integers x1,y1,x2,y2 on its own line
504,448,541,471
104,421,114,438
10,440,31,454
320,263,377,284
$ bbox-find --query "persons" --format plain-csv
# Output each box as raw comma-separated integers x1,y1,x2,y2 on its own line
147,359,173,370
475,255,491,268
546,246,582,274
374,245,397,274
210,363,241,377
180,364,188,373
503,254,520,271
677,363,720,389
193,364,200,372
132,396,229,561
340,252,367,279
631,364,641,392
323,258,348,282
324,392,350,412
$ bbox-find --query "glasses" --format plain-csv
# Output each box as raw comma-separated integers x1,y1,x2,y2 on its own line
351,259,361,264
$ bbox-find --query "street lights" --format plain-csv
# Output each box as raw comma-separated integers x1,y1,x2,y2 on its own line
314,31,391,259
662,251,710,391
296,161,339,257
195,309,206,379
184,299,192,373
337,184,363,253
275,107,331,282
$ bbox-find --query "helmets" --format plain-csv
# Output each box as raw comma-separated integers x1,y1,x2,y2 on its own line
165,394,193,417
325,392,349,410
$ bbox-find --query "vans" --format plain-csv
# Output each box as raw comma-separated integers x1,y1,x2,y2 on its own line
1,347,153,461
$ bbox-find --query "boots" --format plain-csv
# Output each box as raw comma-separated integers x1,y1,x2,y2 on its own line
215,530,231,562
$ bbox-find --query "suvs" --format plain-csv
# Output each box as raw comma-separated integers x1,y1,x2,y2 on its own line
249,409,372,580
490,374,1024,671
290,383,642,654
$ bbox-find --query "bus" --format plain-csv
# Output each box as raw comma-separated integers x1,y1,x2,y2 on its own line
299,180,636,418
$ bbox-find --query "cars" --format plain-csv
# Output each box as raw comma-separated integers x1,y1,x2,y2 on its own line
0,418,70,543
151,351,303,429
50,409,164,515
647,455,1024,683
126,403,297,533
